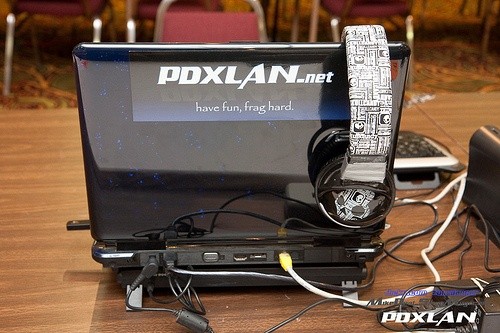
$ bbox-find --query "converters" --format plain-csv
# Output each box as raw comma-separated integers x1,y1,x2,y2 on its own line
431,277,500,332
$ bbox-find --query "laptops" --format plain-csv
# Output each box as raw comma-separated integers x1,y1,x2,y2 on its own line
74,41,412,269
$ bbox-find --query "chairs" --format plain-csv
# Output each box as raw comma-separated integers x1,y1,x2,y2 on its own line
122,0,230,44
313,0,419,90
2,0,117,98
151,0,270,47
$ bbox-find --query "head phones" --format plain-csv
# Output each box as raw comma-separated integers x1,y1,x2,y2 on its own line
316,23,395,229
307,59,350,188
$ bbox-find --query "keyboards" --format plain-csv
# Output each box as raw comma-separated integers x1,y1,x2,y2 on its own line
394,130,467,191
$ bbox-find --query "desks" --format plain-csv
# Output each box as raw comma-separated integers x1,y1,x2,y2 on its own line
402,86,499,156
0,91,500,331
